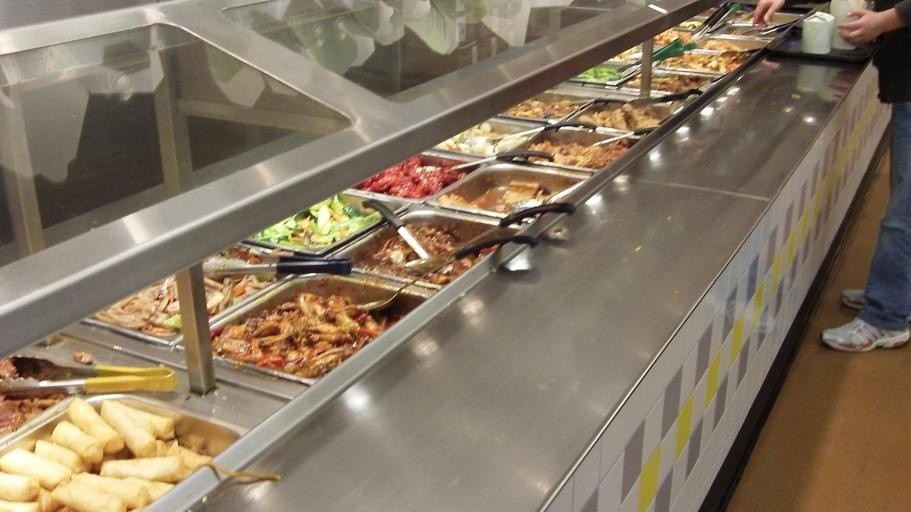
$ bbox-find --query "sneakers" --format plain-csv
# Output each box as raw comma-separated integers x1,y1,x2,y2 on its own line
841,288,867,309
820,316,910,352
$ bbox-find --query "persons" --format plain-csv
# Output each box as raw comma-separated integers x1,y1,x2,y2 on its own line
752,0,911,354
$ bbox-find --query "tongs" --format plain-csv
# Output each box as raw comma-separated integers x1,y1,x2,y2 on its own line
203,240,352,280
679,0,734,49
743,21,800,38
612,38,682,79
1,351,178,403
